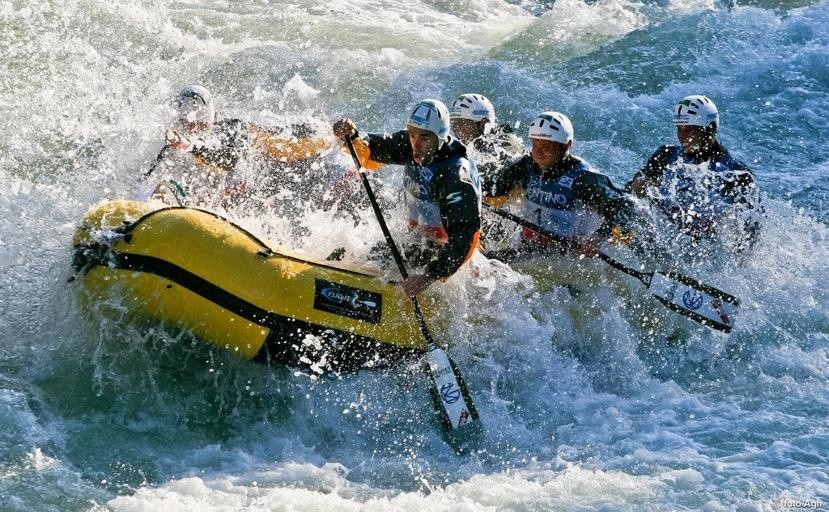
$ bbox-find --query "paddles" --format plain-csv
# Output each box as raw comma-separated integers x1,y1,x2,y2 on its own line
344,132,482,449
491,205,738,331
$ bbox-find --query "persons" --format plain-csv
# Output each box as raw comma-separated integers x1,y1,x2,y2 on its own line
476,110,638,262
626,91,768,274
144,87,382,184
331,97,484,298
452,90,524,261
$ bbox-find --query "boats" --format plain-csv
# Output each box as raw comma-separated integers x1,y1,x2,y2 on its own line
72,195,723,377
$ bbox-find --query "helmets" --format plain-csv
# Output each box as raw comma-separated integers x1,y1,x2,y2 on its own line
673,95,720,128
529,111,574,146
407,99,450,141
450,94,495,124
178,85,213,125
